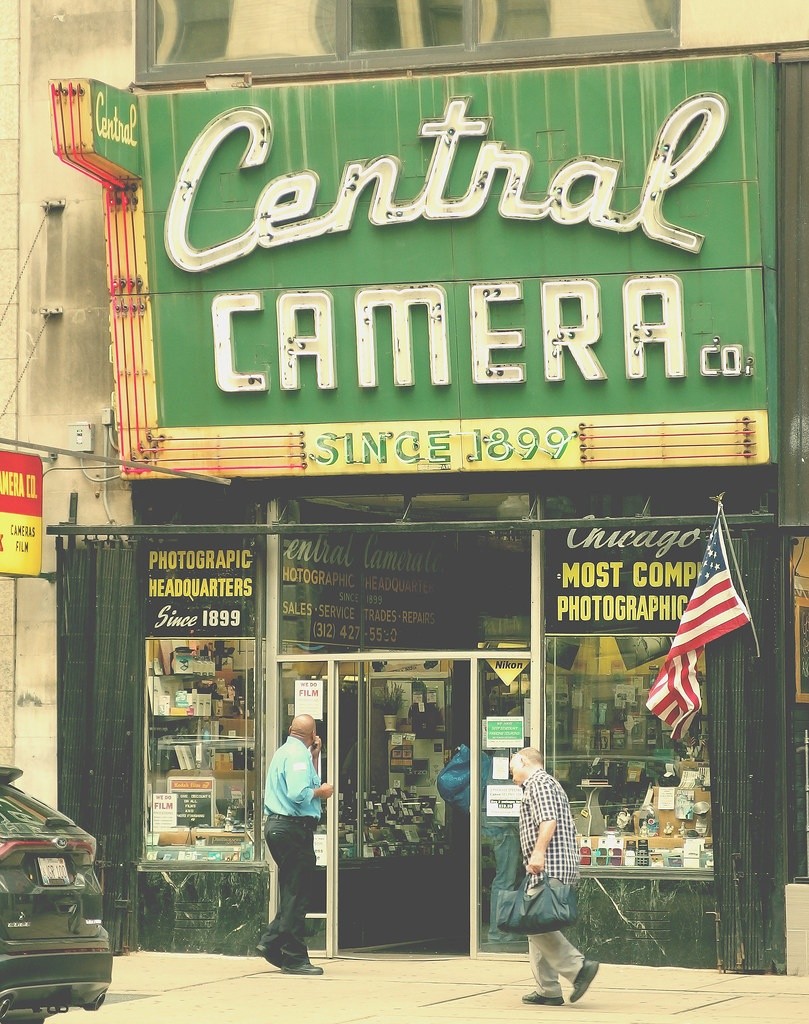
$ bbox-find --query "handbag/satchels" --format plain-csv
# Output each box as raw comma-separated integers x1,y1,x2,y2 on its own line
437,743,491,817
496,869,579,936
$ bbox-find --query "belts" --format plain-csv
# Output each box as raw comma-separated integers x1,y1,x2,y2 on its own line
270,814,318,824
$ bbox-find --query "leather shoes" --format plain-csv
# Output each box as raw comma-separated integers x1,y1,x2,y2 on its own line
570,960,599,1003
280,963,323,975
522,991,564,1006
256,945,283,968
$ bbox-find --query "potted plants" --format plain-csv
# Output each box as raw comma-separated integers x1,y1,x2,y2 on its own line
372,679,405,731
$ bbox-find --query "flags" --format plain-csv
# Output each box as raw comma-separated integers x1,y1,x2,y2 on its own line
646,502,752,739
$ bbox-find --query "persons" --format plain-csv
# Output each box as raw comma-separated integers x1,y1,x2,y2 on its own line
341,709,388,792
509,747,600,1006
480,707,528,943
256,714,333,975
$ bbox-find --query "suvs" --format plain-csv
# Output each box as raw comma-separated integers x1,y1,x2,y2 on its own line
0,763,114,1024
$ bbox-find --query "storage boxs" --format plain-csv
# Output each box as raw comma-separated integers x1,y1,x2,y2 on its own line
147,639,254,770
